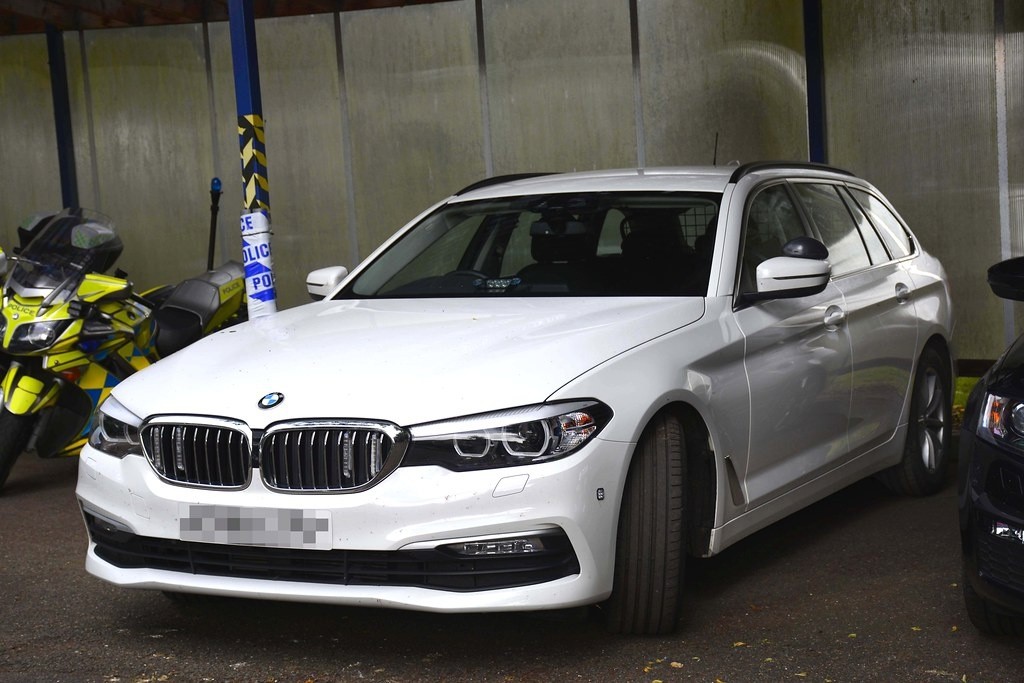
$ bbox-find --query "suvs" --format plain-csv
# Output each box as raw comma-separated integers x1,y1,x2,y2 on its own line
76,160,955,637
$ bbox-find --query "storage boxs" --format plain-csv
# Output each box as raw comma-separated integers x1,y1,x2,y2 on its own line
17,215,124,283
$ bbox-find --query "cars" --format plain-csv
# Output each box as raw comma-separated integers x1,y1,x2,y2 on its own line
956,258,1024,646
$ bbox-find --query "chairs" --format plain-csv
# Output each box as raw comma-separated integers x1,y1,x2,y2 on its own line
500,214,604,296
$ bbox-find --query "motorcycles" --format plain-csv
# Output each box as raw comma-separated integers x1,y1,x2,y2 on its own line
0,175,246,491
